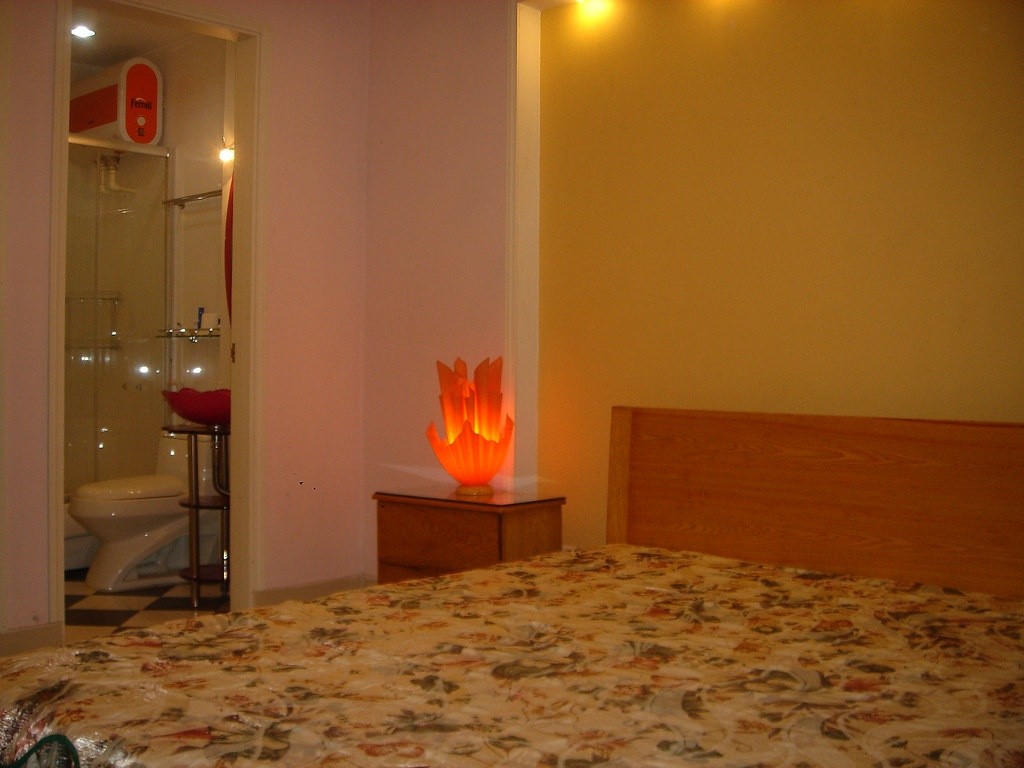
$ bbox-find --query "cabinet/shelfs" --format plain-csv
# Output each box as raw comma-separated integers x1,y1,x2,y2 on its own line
161,423,233,610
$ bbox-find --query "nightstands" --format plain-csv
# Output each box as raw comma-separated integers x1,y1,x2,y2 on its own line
371,489,567,585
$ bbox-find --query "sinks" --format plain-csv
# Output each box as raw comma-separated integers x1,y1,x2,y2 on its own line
161,388,233,424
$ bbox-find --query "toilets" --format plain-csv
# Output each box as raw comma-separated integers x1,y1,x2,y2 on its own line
68,423,223,594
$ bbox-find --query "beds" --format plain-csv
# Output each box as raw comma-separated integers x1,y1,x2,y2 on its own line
0,405,1024,768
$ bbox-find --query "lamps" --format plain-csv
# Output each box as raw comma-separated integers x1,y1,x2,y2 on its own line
426,356,514,496
